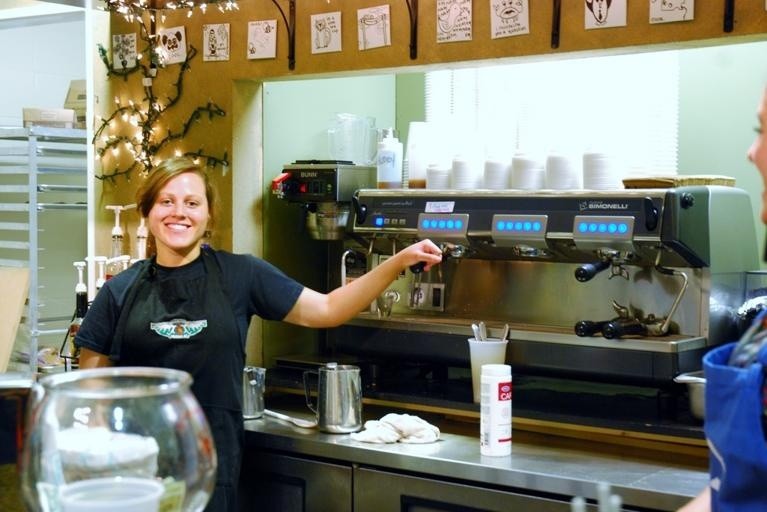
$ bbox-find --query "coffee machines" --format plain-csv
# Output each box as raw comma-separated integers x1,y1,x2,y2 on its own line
337,178,758,380
272,158,377,375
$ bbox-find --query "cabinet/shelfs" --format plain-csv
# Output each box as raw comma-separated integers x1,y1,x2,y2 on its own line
1,123,89,390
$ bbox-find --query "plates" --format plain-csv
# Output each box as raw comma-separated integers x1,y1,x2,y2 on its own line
424,151,627,192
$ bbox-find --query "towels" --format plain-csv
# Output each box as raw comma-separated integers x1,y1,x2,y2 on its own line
350,413,440,444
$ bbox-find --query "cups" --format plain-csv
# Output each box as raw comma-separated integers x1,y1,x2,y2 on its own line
402,66,485,188
465,337,510,403
55,476,166,512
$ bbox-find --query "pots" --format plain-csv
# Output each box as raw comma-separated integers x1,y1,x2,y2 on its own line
672,367,706,419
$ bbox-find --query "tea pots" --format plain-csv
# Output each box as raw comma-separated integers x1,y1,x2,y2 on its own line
303,364,366,434
241,364,268,419
328,114,378,165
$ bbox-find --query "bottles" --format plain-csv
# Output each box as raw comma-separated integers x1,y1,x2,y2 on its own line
479,363,512,455
373,124,403,191
18,367,220,512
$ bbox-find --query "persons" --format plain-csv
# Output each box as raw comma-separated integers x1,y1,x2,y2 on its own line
675,86,767,511
72,159,443,512
